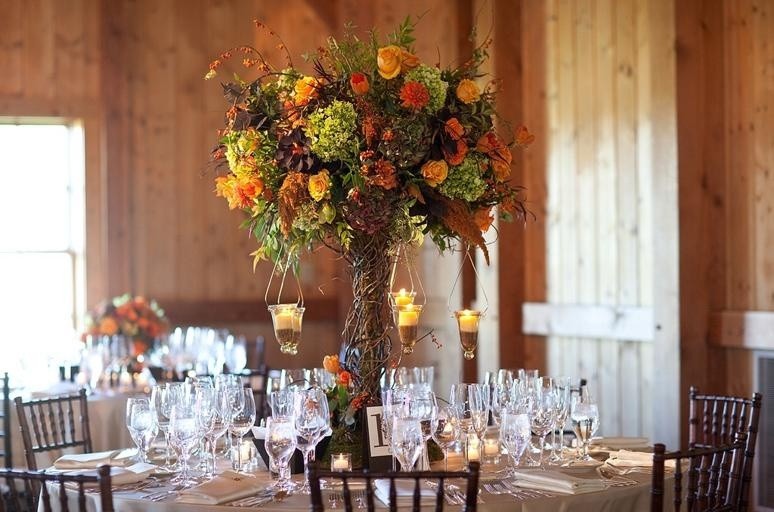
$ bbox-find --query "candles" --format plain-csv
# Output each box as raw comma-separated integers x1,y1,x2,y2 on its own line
272,303,295,330
459,309,478,332
395,288,410,305
399,304,418,326
294,309,302,330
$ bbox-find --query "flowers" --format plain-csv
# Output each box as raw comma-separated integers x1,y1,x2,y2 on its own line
203,13,534,472
81,294,171,385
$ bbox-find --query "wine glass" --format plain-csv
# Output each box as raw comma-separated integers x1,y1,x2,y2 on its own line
261,364,332,489
44,325,248,396
382,366,599,490
128,364,255,491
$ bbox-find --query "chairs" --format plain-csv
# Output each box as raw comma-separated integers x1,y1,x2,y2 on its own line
650,433,747,512
307,460,480,512
0,464,115,512
14,388,93,472
688,386,763,512
